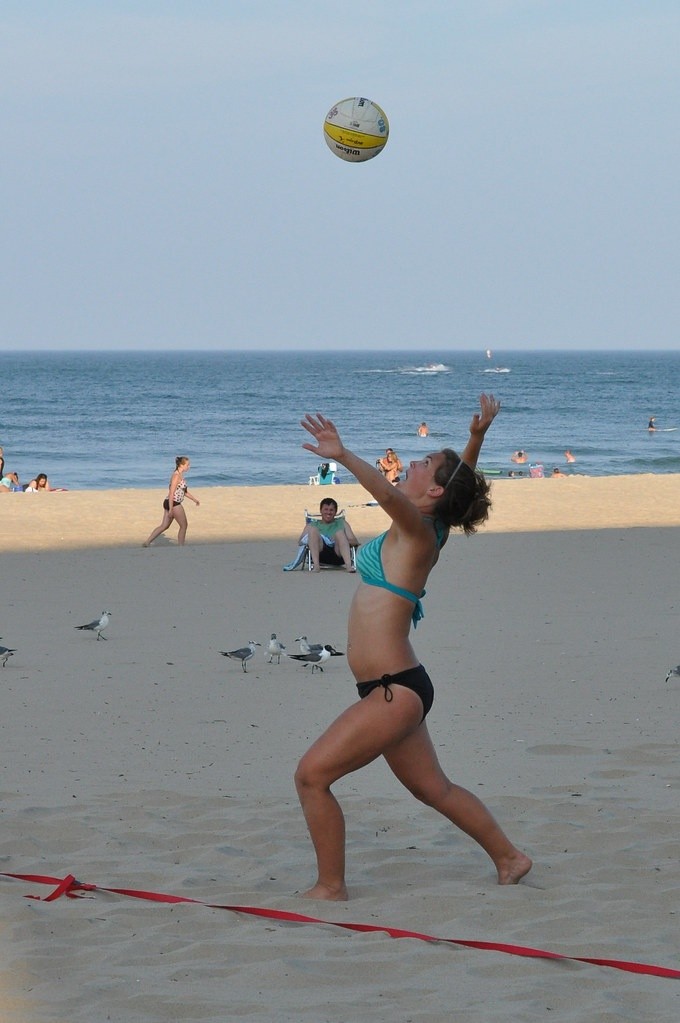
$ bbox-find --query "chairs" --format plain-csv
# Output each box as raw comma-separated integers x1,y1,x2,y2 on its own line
309,463,336,486
304,508,357,572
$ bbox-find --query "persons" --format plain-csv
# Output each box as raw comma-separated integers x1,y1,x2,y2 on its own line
551,468,567,479
378,447,403,483
0,472,19,493
0,447,4,481
292,393,533,901
297,497,359,573
508,470,514,477
565,451,575,462
511,452,529,463
142,456,200,547
29,473,49,491
648,417,656,431
418,422,428,437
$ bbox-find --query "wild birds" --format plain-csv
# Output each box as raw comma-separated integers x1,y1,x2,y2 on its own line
75,611,115,641
217,632,344,675
0,640,20,671
665,665,680,683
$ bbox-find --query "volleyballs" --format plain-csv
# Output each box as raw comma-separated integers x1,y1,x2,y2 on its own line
324,95,391,163
